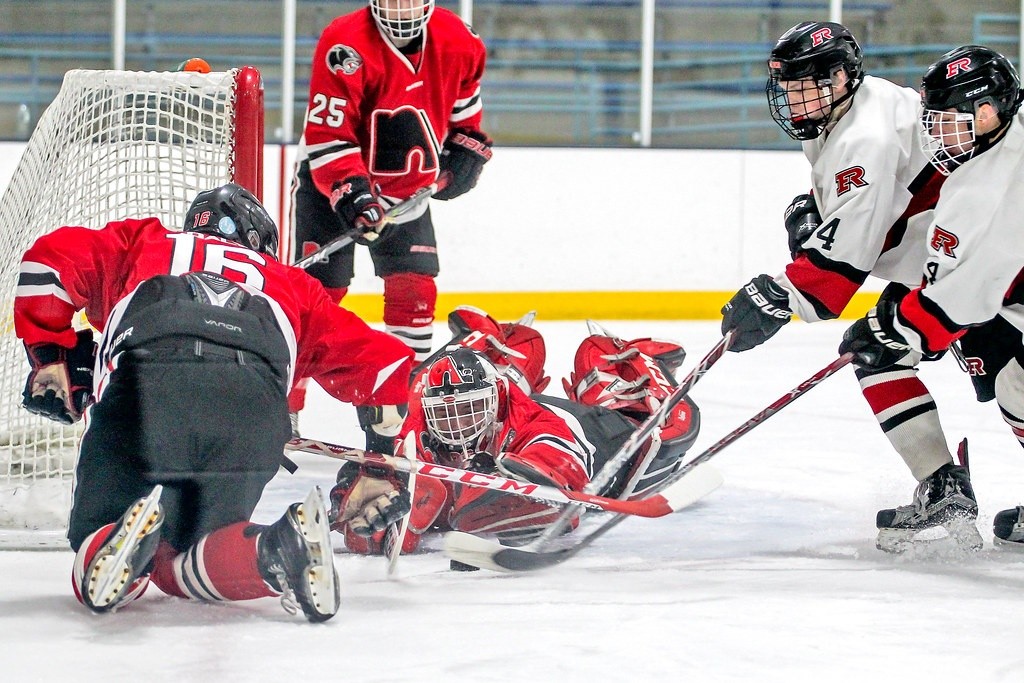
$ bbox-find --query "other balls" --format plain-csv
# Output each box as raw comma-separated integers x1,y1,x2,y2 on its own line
450,560,480,572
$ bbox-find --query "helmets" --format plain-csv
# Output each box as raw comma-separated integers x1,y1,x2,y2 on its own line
918,45,1020,176
420,346,498,465
369,0,435,47
765,21,865,141
183,184,279,262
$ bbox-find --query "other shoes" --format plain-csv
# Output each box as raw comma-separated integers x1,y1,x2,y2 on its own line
629,336,685,369
448,306,503,334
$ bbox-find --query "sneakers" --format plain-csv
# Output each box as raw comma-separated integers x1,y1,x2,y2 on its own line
244,486,340,623
82,485,165,611
876,437,984,552
993,506,1024,549
288,410,300,438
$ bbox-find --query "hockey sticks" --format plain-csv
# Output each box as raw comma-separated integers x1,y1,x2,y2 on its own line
30,382,723,518
290,175,452,271
489,351,857,571
444,326,743,573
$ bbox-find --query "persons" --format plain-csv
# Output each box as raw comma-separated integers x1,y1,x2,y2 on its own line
721,21,1024,556
13,183,416,623
286,0,494,450
330,305,700,554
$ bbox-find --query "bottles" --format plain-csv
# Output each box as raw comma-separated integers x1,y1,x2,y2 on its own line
169,58,212,73
15,105,30,140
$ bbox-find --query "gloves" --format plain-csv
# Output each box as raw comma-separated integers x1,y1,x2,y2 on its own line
431,128,493,200
19,329,98,424
838,282,913,371
327,460,410,537
721,275,793,352
784,194,822,261
331,171,384,248
